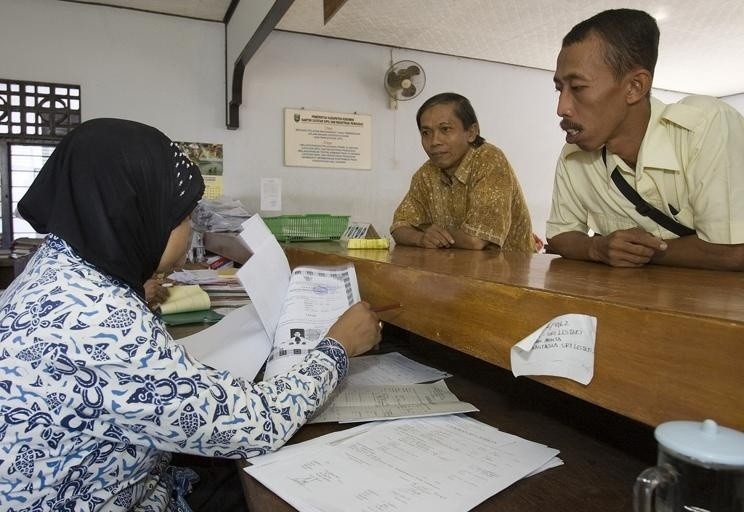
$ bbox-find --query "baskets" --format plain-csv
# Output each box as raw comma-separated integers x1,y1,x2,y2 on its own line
261,213,350,244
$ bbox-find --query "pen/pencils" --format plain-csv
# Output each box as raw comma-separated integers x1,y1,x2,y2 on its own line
368,304,404,313
160,283,174,288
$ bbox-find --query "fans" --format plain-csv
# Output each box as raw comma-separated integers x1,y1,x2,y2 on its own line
384,58,426,102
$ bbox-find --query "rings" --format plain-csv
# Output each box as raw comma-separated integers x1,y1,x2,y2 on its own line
379,320,384,328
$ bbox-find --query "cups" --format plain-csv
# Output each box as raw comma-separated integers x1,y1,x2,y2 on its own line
633,419,744,512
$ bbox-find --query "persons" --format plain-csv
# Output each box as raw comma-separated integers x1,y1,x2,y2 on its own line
547,9,744,271
143,279,170,308
390,92,536,254
0,117,382,512
